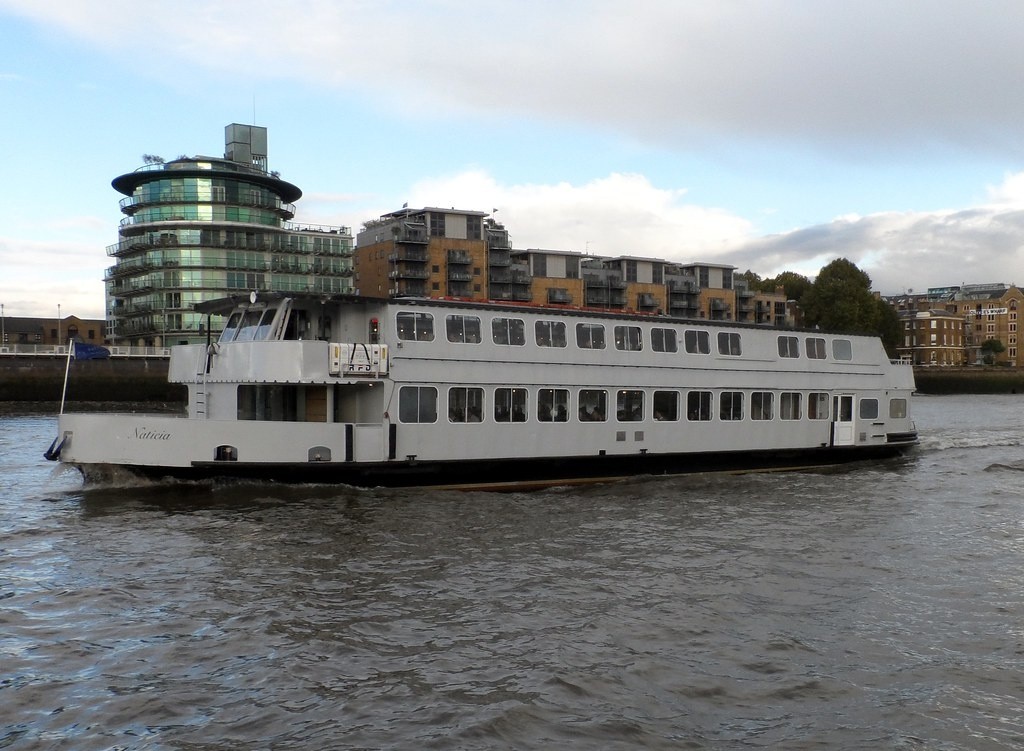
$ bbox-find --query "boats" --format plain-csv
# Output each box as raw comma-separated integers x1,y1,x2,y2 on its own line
43,285,922,494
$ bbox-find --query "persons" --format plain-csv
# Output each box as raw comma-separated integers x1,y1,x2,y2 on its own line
397,320,740,356
447,403,741,421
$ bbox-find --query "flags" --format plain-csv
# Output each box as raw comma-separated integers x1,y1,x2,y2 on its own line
74,341,111,363
